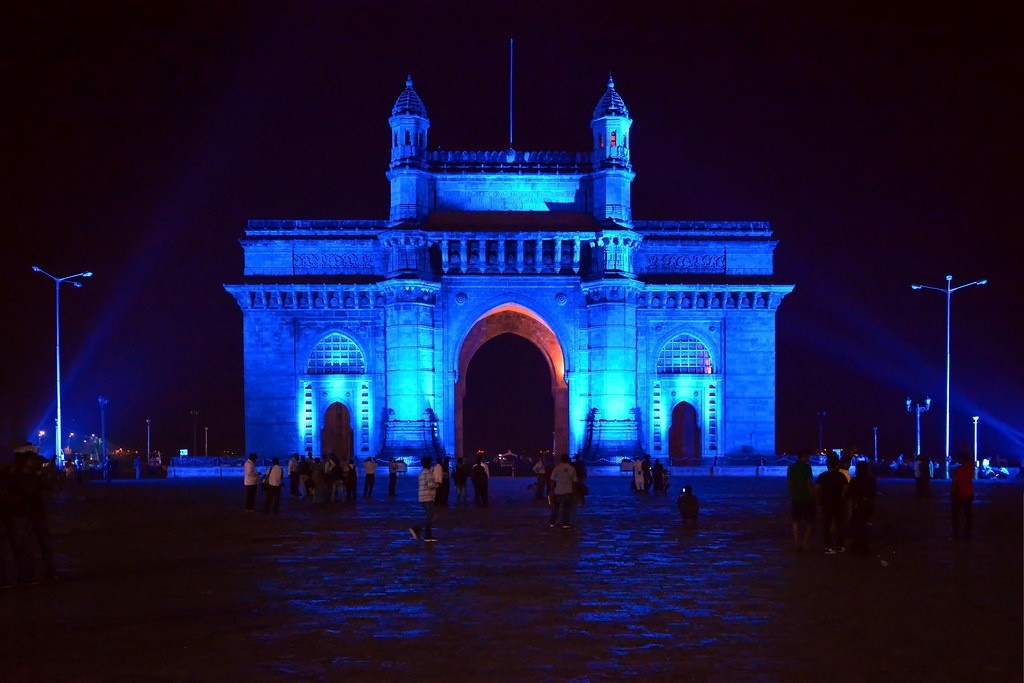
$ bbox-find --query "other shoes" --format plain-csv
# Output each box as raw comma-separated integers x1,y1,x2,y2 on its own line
825,547,837,554
409,527,420,540
424,537,437,541
550,522,555,527
837,547,846,552
563,524,571,528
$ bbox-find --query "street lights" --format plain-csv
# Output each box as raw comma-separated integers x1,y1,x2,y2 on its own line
911,274,987,480
190,408,201,456
96,394,109,480
31,265,94,472
146,418,151,465
906,396,931,456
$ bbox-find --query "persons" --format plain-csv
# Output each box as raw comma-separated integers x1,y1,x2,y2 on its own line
434,457,490,504
363,456,375,499
947,443,975,542
149,453,158,478
104,455,113,484
914,454,931,495
39,452,78,500
631,453,671,497
242,451,359,511
786,449,882,552
527,455,587,529
388,457,399,497
409,456,441,542
3,441,68,624
133,454,144,480
677,485,700,521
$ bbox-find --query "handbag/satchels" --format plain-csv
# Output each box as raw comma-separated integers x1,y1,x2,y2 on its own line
263,477,269,484
